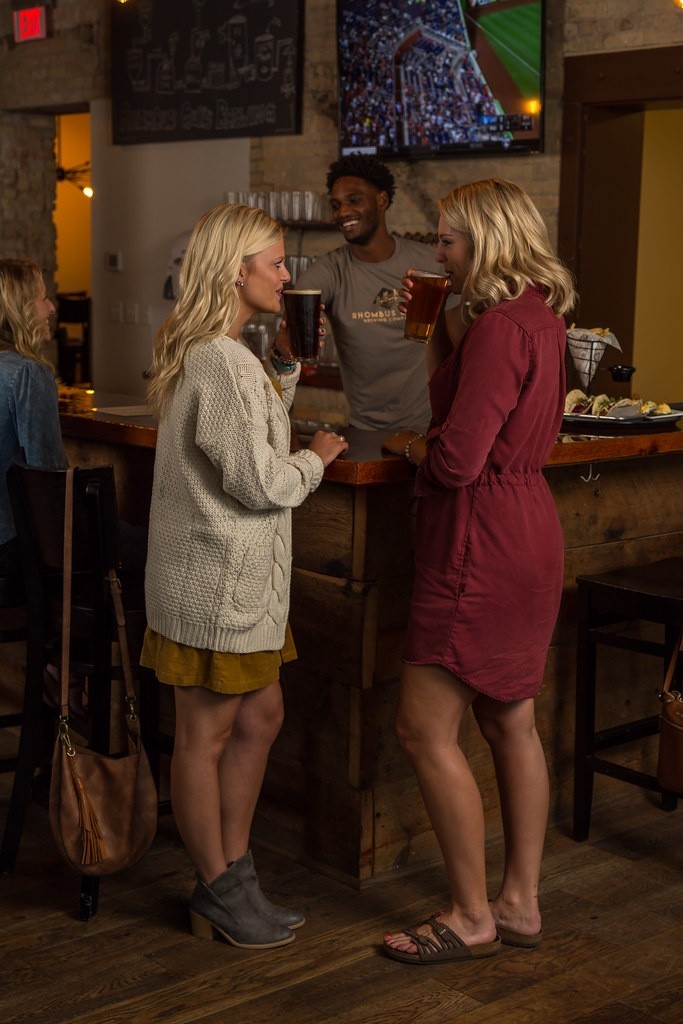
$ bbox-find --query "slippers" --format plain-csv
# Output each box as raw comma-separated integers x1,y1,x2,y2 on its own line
430,897,544,947
384,916,502,963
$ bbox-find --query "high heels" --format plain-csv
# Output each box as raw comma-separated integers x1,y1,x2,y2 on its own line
232,848,306,929
190,860,296,948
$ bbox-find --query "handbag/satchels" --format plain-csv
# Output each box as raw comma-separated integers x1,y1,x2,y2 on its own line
51,716,159,875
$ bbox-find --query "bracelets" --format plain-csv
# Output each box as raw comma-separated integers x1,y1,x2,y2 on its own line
406,434,426,467
267,343,298,373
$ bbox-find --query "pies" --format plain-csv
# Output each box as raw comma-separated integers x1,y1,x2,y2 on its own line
564,389,632,417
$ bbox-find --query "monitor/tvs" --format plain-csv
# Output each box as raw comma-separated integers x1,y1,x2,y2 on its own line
337,0,547,162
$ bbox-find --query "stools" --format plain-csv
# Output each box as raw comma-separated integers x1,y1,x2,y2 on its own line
570,557,682,839
0,463,158,922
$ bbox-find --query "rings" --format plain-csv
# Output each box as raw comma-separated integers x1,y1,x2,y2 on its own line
339,436,344,442
331,432,337,437
394,432,399,438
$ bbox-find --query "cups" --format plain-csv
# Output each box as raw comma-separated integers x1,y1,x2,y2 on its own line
283,289,322,362
284,253,321,286
225,191,336,224
405,269,448,343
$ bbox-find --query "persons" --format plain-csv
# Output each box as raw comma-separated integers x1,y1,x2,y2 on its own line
275,152,463,432
375,179,565,964
335,0,513,148
139,205,350,949
0,257,144,723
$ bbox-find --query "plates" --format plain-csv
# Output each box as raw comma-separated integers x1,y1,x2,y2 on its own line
561,409,682,426
